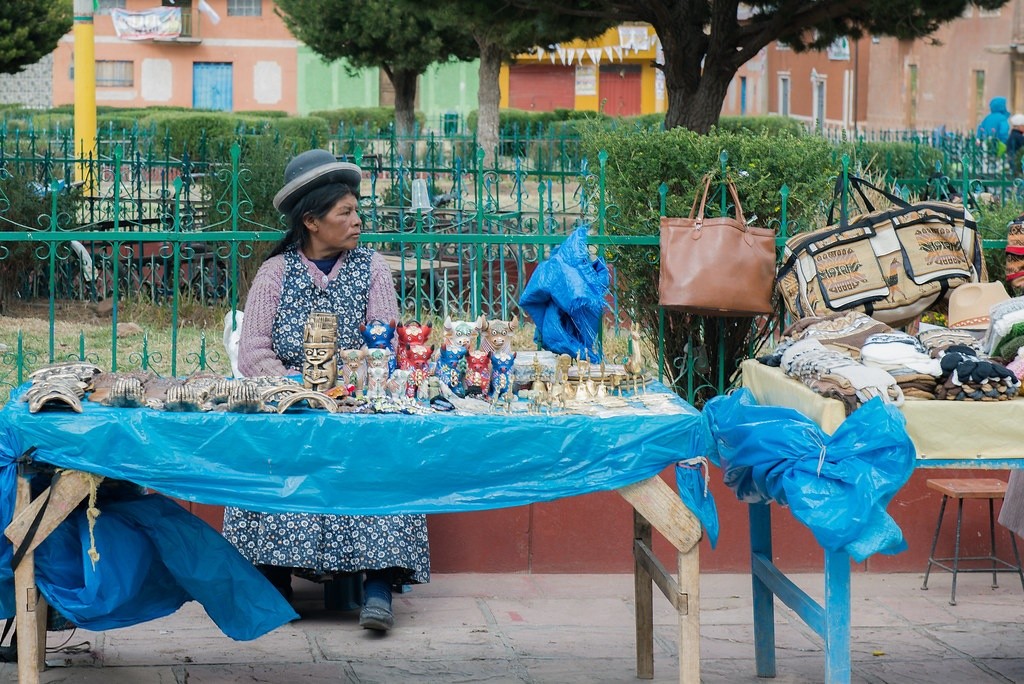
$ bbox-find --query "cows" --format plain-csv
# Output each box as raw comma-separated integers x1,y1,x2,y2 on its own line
400,342,436,397
358,319,398,378
395,318,433,370
433,342,468,399
480,313,520,356
441,315,482,350
338,343,368,399
363,344,392,401
489,352,517,397
461,346,493,404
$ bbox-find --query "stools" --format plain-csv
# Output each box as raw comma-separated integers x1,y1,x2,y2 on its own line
920,479,1024,607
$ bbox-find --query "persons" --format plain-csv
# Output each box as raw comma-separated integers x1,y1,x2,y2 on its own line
299,312,339,407
976,96,1011,174
1006,114,1024,175
222,150,429,636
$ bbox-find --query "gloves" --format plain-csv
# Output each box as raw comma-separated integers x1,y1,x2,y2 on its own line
934,344,1021,400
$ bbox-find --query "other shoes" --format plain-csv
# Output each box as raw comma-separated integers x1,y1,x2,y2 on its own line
359,584,396,631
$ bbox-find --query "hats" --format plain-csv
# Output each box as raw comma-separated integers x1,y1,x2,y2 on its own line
273,149,362,215
947,280,1013,330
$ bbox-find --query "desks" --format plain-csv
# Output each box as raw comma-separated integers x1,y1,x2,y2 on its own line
0,377,708,684
741,358,1024,684
381,254,466,319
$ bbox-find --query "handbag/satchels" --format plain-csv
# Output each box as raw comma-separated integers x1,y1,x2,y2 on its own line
771,170,990,324
658,169,776,319
